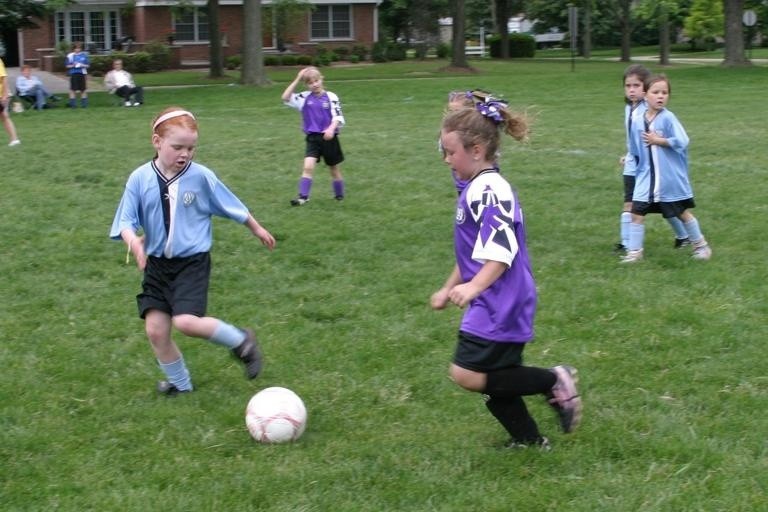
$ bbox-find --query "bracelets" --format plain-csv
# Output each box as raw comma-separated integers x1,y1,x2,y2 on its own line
125,233,137,267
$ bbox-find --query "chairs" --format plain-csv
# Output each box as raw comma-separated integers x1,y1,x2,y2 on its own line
108,89,135,106
17,91,39,111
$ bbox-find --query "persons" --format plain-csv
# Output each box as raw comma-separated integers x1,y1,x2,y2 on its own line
14,65,63,110
0,57,20,147
437,85,512,198
618,74,714,263
612,64,690,255
280,65,346,205
63,40,91,108
426,100,584,453
104,58,144,107
104,108,278,396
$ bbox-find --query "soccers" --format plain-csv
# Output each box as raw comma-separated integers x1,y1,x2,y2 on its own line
244,385,307,443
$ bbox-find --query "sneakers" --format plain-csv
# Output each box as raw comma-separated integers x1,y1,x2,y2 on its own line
506,436,551,453
51,96,62,102
290,195,344,206
611,243,643,263
547,364,581,434
158,381,188,395
125,100,140,106
232,326,262,379
674,235,712,260
